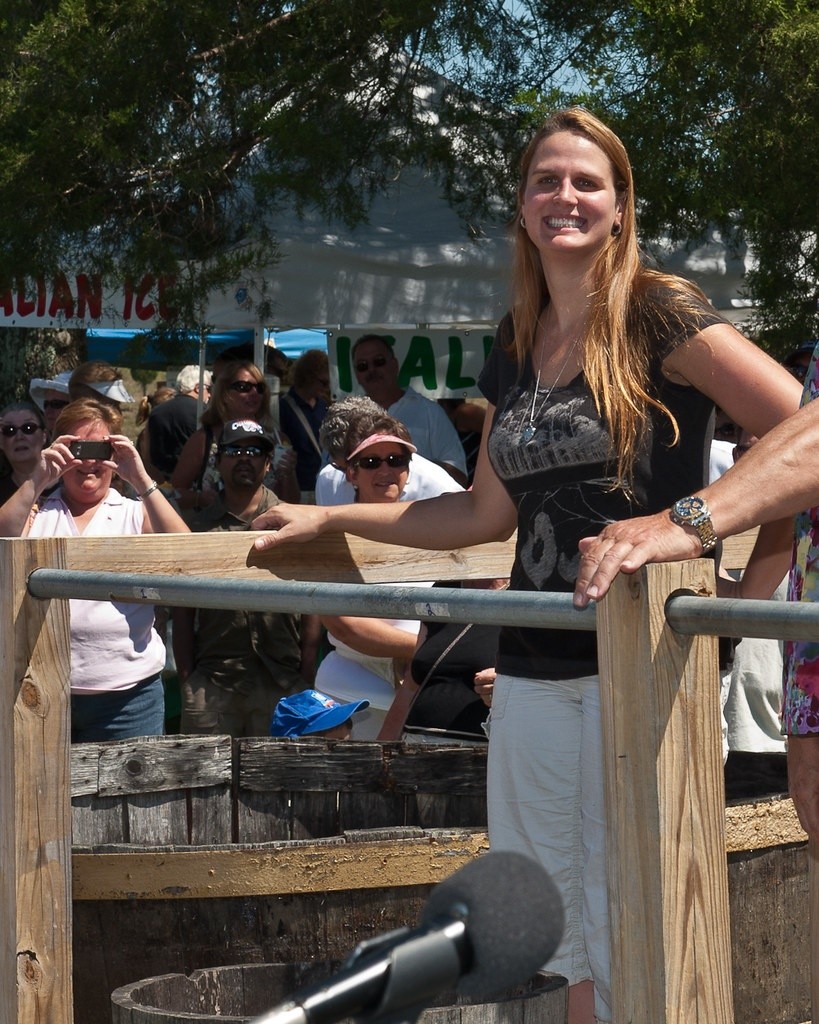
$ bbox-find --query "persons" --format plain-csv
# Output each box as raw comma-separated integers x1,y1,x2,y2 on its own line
0,334,818,859
251,111,804,1021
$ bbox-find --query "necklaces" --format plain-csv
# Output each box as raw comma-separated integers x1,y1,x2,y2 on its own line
523,303,585,442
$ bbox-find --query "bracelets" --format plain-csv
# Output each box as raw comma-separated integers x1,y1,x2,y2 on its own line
135,479,159,500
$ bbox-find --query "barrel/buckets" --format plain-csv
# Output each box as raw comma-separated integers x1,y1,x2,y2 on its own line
113,960,571,1024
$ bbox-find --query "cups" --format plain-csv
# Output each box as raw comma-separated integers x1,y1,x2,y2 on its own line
274,444,292,470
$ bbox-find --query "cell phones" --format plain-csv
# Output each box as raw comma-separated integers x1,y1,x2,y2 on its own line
70,439,113,459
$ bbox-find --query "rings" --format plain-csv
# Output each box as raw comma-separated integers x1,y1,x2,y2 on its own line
489,694,492,700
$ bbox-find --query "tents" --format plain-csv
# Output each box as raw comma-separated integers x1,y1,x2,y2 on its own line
0,36,819,450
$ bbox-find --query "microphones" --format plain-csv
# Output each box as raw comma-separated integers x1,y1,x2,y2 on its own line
247,849,566,1024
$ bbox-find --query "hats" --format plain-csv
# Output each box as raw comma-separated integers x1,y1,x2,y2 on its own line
71,377,136,403
346,432,417,460
218,418,276,458
29,372,73,410
269,689,370,739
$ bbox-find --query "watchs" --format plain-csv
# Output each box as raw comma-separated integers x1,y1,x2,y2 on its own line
670,495,718,554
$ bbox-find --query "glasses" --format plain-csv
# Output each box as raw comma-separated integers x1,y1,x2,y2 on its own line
353,355,387,373
314,376,330,388
43,399,70,408
1,422,41,436
204,384,212,393
354,453,408,469
221,446,269,458
226,380,267,395
